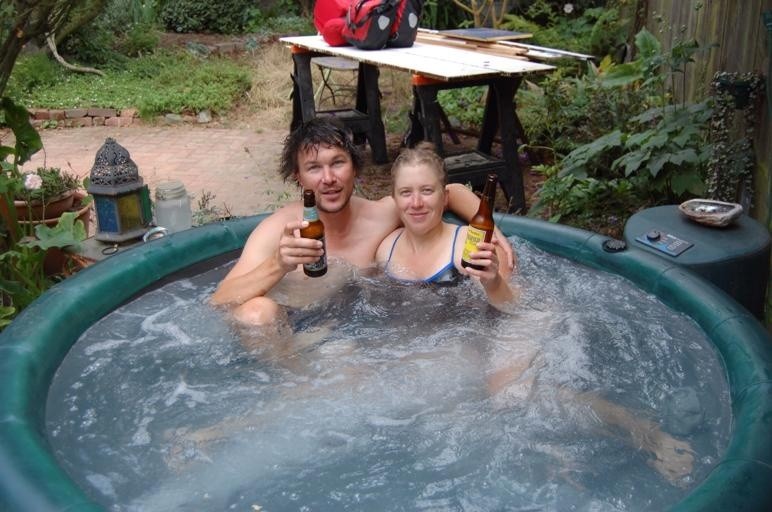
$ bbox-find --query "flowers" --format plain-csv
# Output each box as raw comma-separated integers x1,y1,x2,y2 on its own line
11,169,85,201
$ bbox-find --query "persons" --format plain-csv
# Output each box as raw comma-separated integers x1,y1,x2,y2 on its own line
374,141,694,490
163,115,516,475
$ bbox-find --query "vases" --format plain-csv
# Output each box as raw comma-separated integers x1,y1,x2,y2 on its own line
16,192,93,277
9,188,78,220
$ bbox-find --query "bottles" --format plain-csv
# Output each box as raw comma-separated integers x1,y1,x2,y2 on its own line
299,187,330,279
156,180,192,234
460,171,499,271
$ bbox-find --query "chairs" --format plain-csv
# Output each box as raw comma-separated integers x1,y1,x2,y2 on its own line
311,29,369,115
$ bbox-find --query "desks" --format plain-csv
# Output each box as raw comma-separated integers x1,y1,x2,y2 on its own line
278,26,597,213
